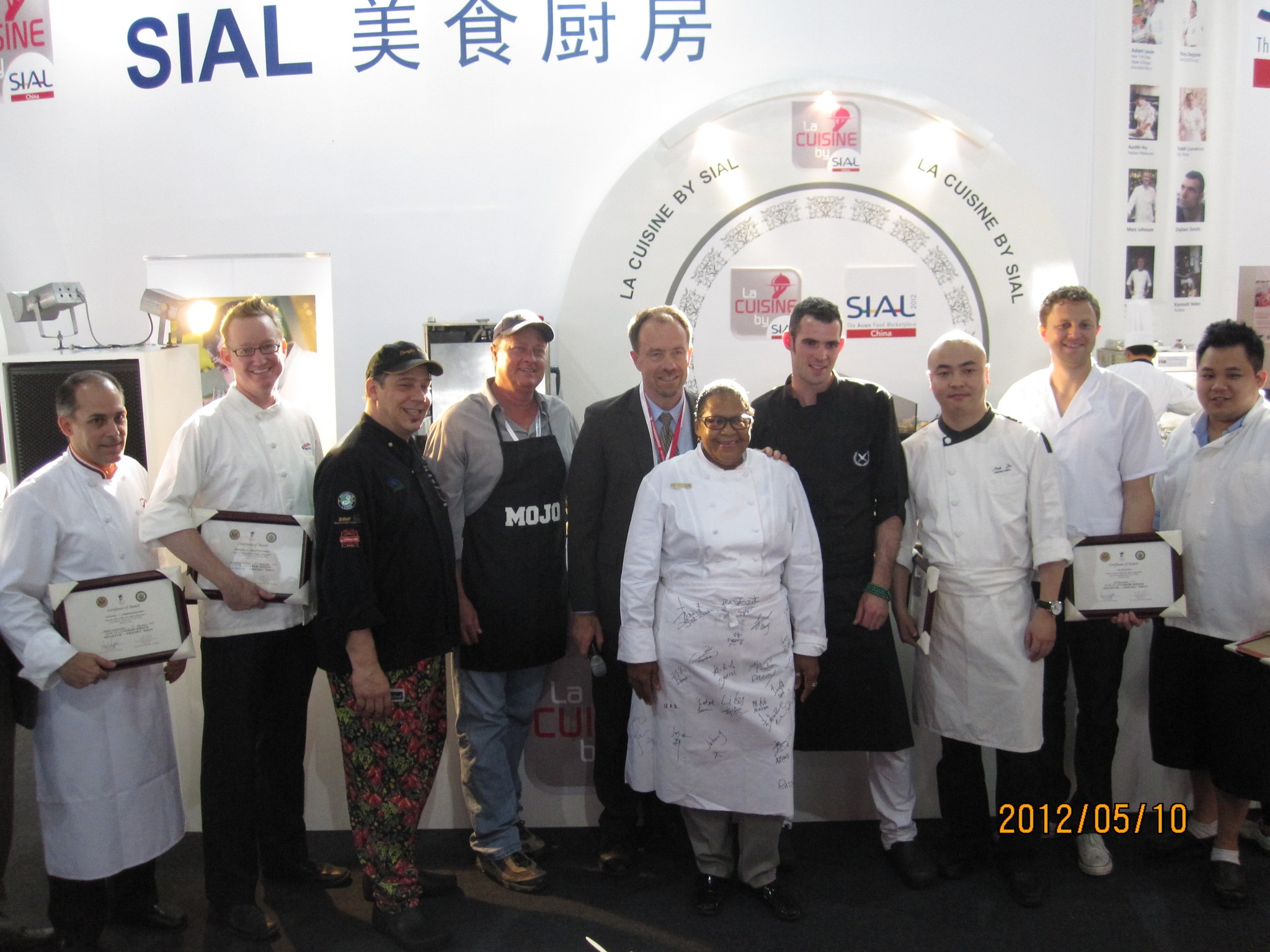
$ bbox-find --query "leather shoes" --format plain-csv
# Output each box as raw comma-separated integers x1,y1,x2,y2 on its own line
694,874,720,915
290,859,350,884
208,903,279,939
740,879,801,920
145,905,185,932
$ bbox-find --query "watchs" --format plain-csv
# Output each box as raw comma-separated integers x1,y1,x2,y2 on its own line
1036,599,1062,616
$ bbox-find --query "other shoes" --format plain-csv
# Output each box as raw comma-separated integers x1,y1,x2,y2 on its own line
1208,847,1242,888
373,904,448,948
600,837,626,865
1003,847,1042,906
364,869,457,901
1175,830,1219,846
888,840,939,885
24,926,57,947
941,830,992,879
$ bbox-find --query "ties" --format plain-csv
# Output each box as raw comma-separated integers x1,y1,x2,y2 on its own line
658,412,680,460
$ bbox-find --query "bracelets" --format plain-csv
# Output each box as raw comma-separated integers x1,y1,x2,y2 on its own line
864,583,892,601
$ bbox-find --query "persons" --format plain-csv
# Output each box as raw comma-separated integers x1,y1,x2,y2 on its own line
424,308,591,894
1153,319,1269,894
1132,93,1157,139
205,299,316,417
1102,300,1203,426
1181,93,1204,141
311,341,466,942
139,296,350,940
614,380,828,919
0,375,193,952
1182,0,1200,46
1126,256,1195,299
566,306,789,874
1127,170,1156,223
746,299,942,885
1142,0,1166,43
1176,172,1205,222
890,330,1074,906
999,286,1166,877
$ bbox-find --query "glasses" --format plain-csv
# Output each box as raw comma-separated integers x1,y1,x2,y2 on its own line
697,416,752,430
228,340,280,357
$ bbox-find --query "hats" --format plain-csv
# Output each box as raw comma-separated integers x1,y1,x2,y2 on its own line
366,340,443,378
493,310,554,343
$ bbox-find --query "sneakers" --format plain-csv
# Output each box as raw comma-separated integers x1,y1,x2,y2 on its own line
1076,833,1113,876
517,820,548,861
476,850,550,893
